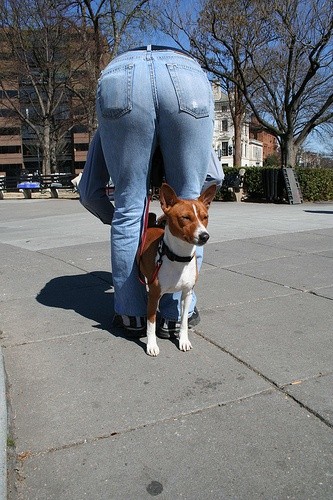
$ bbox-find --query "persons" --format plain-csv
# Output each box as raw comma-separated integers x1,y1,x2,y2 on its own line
78,44,224,338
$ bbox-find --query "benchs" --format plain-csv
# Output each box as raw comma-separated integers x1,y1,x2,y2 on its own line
0,169,246,202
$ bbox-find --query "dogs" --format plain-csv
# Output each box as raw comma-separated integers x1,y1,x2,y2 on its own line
136,182,219,358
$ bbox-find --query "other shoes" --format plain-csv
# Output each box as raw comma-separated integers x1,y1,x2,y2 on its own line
156,306,200,339
122,315,147,331
